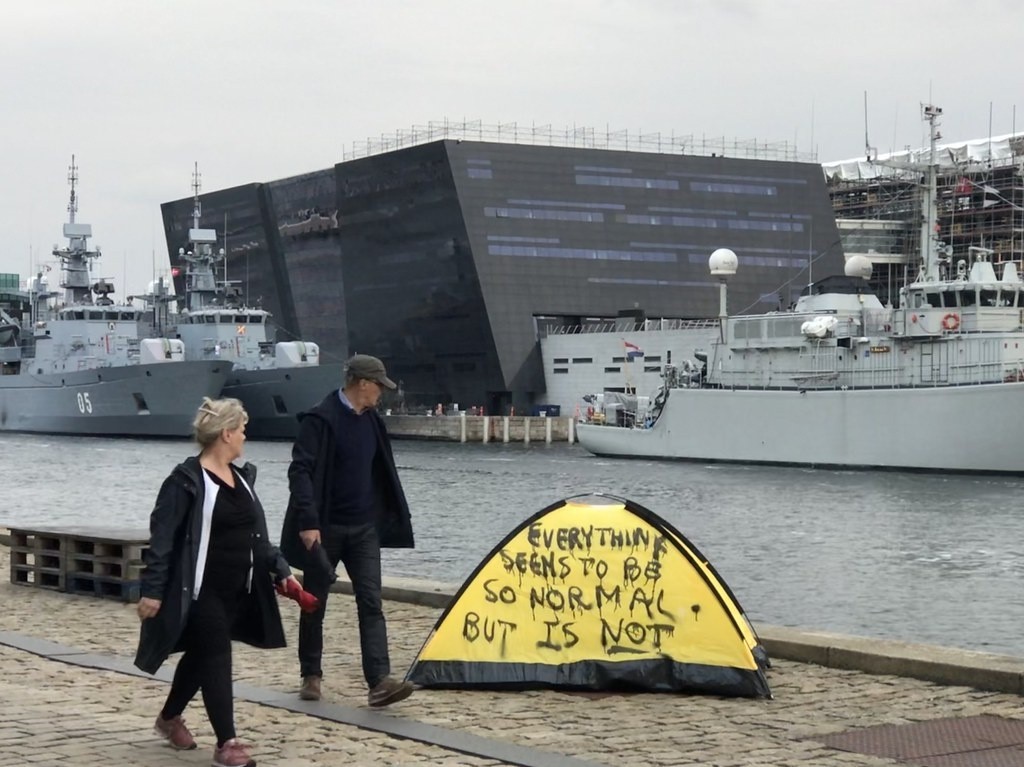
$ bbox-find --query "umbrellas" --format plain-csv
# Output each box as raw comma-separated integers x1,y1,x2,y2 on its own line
274,579,320,613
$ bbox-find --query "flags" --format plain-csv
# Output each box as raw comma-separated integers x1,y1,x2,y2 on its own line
625,342,644,358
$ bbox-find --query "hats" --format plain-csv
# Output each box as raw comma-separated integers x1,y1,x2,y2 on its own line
343,354,397,389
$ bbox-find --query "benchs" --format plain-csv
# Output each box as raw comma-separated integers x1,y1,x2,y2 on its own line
6,527,151,604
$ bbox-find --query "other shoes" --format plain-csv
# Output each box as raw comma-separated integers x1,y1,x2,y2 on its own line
368,677,414,707
300,675,324,699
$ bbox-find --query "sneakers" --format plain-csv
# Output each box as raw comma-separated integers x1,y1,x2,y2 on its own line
211,737,256,767
154,714,197,750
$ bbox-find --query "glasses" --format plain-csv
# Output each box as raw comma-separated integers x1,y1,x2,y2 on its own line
359,377,383,388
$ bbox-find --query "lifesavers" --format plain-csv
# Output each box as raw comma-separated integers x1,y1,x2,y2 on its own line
942,312,960,331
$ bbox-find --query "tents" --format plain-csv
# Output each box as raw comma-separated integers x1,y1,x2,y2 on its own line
403,492,775,701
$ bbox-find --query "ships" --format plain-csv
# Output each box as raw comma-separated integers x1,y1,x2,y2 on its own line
575,78,1024,474
0,153,346,443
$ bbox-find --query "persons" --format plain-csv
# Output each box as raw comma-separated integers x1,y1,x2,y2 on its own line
279,355,416,707
133,395,303,767
